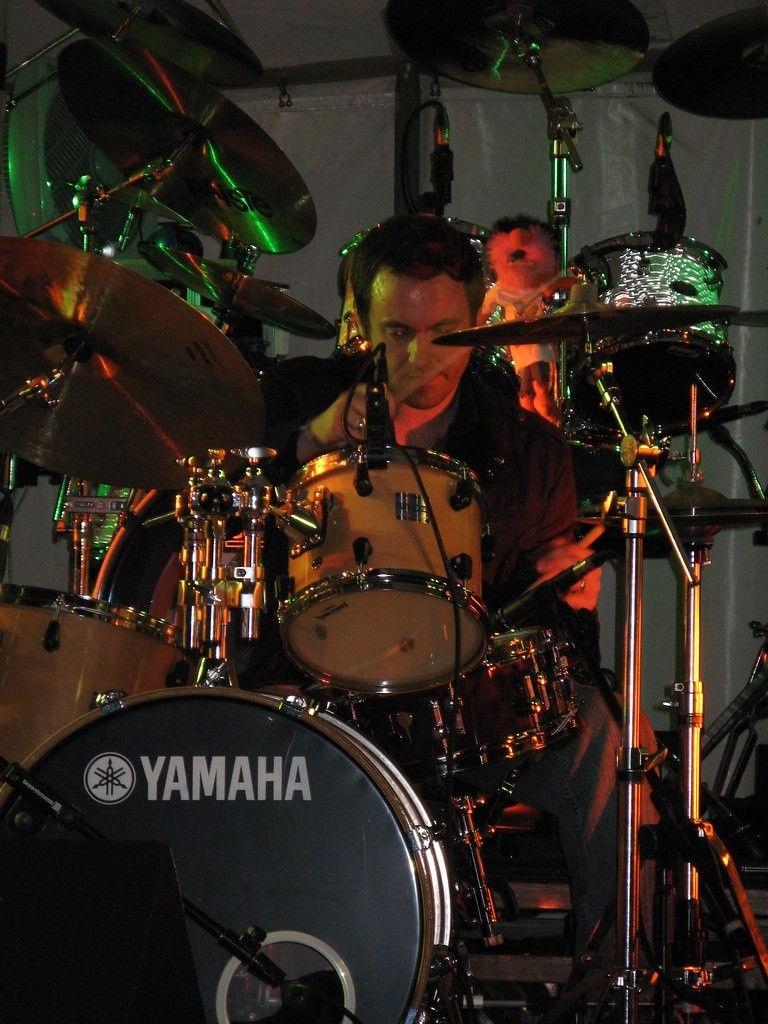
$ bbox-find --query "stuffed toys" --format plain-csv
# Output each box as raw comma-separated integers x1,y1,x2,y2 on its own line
471,215,577,429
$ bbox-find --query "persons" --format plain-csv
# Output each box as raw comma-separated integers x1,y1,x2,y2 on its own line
258,213,651,1024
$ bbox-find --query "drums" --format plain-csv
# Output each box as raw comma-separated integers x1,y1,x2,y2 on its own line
0,680,455,1023
281,443,485,696
1,464,196,652
0,583,197,790
300,623,586,787
563,232,736,433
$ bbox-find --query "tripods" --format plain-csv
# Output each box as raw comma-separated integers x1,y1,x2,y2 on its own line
528,317,768,1024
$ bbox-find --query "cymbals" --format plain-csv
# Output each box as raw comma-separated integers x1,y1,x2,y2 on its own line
143,240,341,342
729,310,768,329
35,1,265,90
570,485,768,545
432,303,737,347
381,0,652,95
59,37,316,255
652,6,768,119
0,236,268,490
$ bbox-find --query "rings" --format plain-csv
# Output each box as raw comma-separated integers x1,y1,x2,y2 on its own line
581,578,585,591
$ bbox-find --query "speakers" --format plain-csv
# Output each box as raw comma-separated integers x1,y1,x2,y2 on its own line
0,835,207,1024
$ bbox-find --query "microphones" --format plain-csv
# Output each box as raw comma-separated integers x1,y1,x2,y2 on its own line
431,107,454,204
248,984,325,1024
646,111,671,216
377,355,389,397
